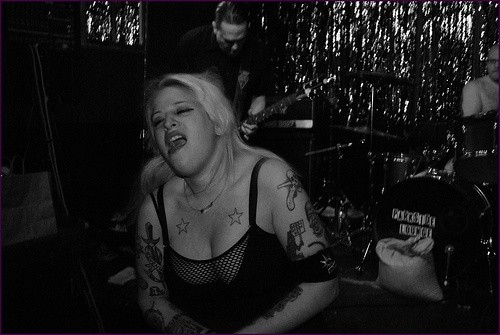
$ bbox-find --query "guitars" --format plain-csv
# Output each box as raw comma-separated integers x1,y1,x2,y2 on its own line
239,72,335,135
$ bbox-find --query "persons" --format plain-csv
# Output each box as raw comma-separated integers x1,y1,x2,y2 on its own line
132,72,344,335
461,42,500,119
163,0,268,142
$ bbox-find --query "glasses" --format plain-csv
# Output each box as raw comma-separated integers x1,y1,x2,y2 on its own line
488,59,500,64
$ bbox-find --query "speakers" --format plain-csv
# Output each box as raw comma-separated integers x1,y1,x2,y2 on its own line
256,134,325,205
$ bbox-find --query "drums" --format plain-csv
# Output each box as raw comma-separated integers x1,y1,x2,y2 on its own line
317,205,364,255
366,151,412,193
371,170,494,283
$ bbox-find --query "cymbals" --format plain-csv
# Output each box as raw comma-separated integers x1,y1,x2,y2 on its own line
347,70,415,87
330,124,397,139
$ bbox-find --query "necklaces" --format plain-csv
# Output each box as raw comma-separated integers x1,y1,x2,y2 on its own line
183,158,230,215
